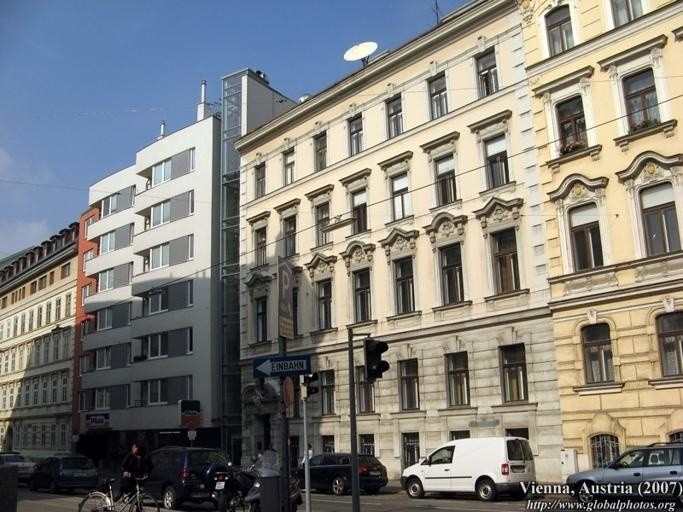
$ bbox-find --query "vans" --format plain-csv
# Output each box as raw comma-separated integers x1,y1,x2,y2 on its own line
399,436,535,500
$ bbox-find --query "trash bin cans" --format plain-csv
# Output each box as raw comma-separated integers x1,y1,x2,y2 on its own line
0,465,17,512
260,477,278,512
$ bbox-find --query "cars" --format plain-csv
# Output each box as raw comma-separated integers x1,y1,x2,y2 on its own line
0,450,38,483
566,441,683,506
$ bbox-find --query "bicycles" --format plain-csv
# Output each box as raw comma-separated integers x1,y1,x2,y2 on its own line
77,471,160,511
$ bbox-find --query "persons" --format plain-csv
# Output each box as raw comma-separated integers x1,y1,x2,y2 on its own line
112,441,150,511
300,443,313,467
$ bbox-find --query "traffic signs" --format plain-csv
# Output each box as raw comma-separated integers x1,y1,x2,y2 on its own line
252,355,311,379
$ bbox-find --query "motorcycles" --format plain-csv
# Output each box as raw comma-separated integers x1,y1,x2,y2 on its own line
245,450,304,511
210,459,255,511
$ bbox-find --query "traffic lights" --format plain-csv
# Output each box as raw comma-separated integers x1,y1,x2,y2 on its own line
363,338,389,381
300,371,320,399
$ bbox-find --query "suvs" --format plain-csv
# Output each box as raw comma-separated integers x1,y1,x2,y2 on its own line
27,453,99,493
295,451,388,496
136,452,260,508
